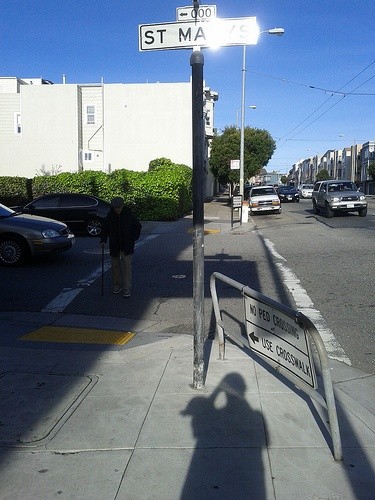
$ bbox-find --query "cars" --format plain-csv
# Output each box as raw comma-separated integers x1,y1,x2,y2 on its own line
299,184,315,198
244,182,279,200
266,183,278,189
8,192,114,238
0,203,75,265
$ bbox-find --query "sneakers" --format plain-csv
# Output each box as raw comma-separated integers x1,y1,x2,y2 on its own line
113,287,122,293
123,290,131,297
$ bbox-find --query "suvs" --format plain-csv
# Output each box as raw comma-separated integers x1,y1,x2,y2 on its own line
249,186,281,215
311,180,368,218
276,185,299,203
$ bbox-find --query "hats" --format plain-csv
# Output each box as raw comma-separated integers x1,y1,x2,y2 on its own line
111,197,124,207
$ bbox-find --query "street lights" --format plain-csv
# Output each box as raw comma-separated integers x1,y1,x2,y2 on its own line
237,105,256,130
234,26,285,199
